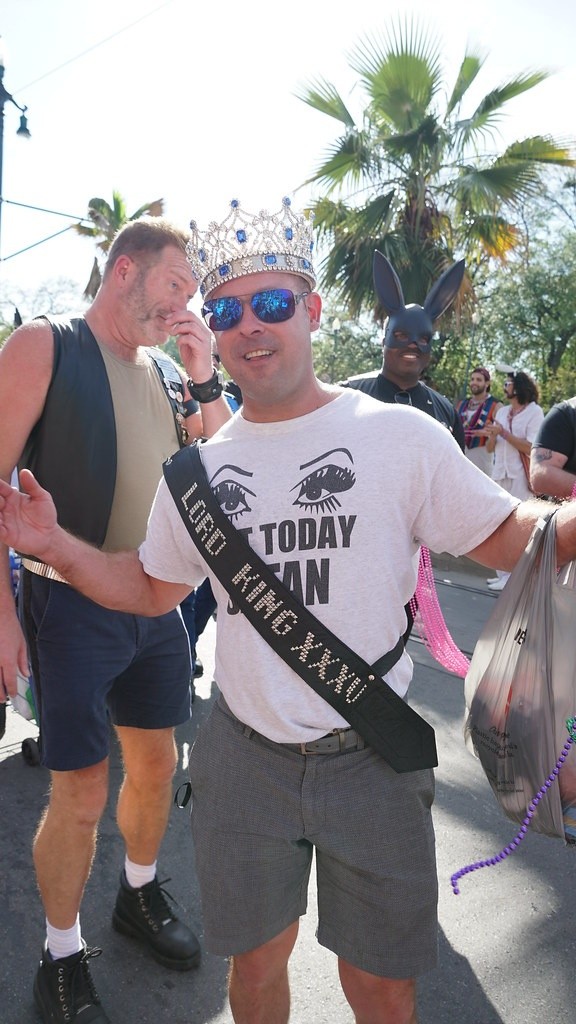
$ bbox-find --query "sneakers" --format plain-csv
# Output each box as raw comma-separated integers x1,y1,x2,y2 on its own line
32,935,113,1024
0,696,40,763
110,868,202,970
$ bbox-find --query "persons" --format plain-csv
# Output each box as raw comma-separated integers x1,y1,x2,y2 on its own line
338,249,466,453
0,199,571,1024
0,215,233,1023
454,368,504,478
529,396,576,504
485,364,544,591
186,353,243,704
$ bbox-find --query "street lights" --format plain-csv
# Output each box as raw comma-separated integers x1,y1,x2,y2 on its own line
0,59,31,223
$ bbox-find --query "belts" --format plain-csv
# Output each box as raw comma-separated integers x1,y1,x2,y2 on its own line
284,729,359,754
21,557,71,585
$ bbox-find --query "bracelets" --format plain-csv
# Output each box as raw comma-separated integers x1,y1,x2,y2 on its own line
187,368,224,403
501,430,508,439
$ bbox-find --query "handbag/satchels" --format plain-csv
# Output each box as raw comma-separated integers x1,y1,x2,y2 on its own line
461,507,576,845
508,408,550,501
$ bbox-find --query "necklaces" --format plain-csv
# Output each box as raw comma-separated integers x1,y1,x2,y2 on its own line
507,403,528,434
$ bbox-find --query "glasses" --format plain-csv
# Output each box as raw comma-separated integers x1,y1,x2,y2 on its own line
503,382,514,389
200,288,309,331
394,390,412,406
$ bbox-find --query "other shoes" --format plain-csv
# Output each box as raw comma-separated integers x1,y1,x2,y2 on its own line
486,575,509,591
194,657,204,674
